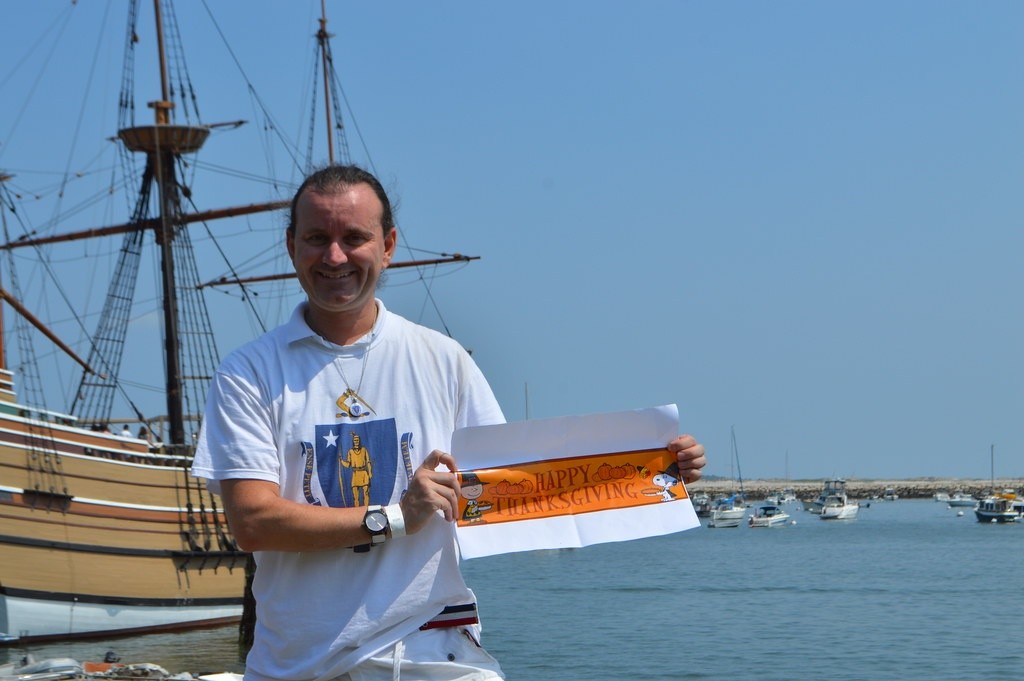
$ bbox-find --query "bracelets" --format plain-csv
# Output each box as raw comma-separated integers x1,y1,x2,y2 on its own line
385,504,407,539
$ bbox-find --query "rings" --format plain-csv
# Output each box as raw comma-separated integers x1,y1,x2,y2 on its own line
439,453,447,463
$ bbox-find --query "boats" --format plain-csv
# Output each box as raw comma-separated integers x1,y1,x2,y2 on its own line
882,488,898,501
933,490,950,502
764,496,781,506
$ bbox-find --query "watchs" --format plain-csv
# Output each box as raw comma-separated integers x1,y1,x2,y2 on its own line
361,505,389,547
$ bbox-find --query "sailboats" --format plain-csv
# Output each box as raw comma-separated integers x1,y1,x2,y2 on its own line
730,425,753,509
780,450,796,503
1,0,483,647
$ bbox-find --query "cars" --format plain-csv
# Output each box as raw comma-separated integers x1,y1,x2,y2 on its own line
974,497,1024,523
802,479,847,514
945,493,978,507
692,492,714,518
708,493,746,528
747,506,790,527
818,494,859,519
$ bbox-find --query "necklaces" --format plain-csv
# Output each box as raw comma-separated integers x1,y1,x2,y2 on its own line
307,306,376,417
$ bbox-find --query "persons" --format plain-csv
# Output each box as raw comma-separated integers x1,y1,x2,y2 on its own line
192,168,705,681
90,422,158,465
